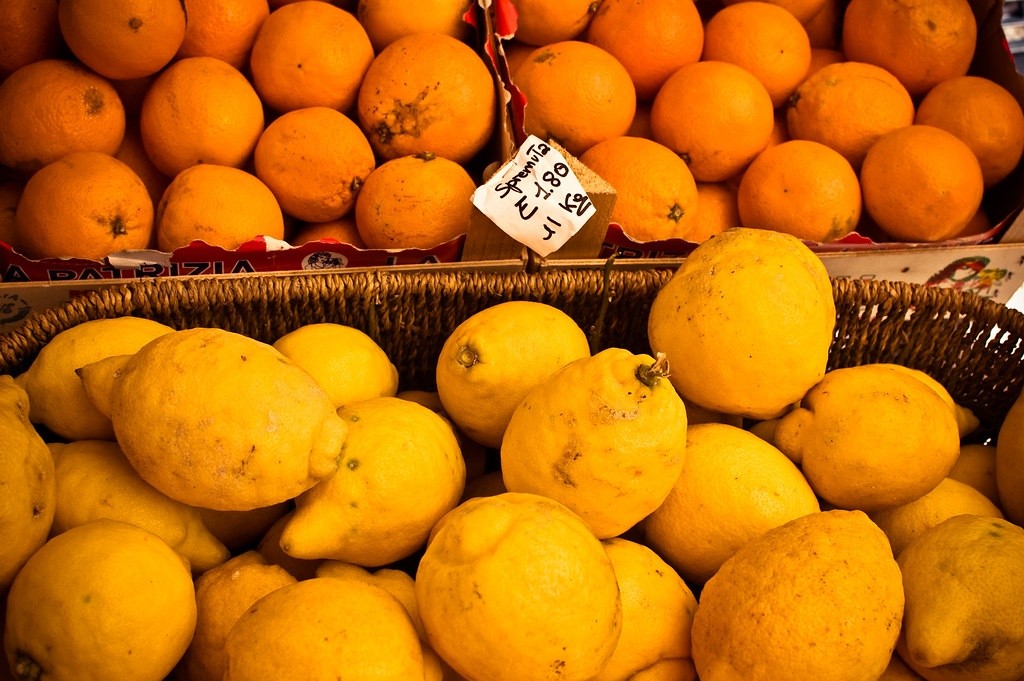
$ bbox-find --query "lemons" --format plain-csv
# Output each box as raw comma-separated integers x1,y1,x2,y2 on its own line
0,226,1024,681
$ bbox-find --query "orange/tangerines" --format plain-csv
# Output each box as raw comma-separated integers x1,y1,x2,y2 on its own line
0,0,497,265
495,0,1024,244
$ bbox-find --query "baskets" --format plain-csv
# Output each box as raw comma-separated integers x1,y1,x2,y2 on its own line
0,269,1024,451
